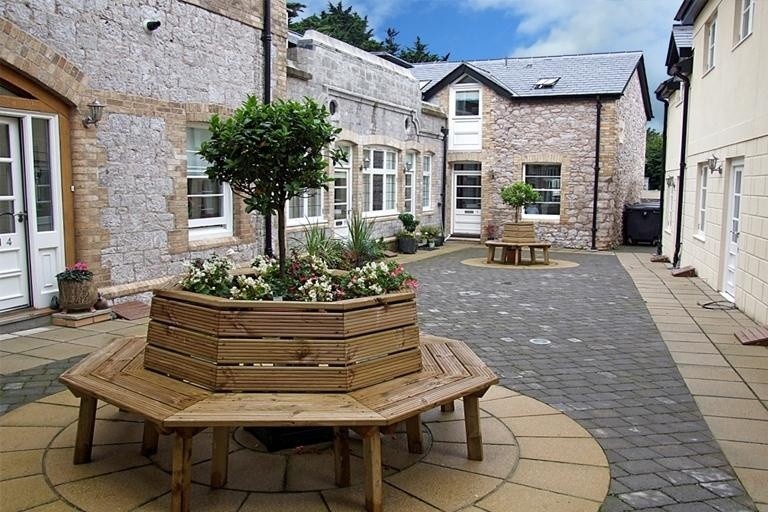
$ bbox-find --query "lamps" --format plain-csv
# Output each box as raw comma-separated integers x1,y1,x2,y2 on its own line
666,174,676,189
707,154,722,175
82,98,105,129
403,161,412,173
359,156,370,172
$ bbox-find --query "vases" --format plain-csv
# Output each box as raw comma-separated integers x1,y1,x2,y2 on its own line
58,279,100,314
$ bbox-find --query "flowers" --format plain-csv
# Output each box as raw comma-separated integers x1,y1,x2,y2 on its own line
55,261,96,284
178,248,420,303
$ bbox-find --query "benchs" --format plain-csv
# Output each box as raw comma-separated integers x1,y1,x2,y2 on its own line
484,239,551,266
56,336,503,511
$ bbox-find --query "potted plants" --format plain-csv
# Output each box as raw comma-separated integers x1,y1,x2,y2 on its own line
397,212,445,254
498,182,541,242
